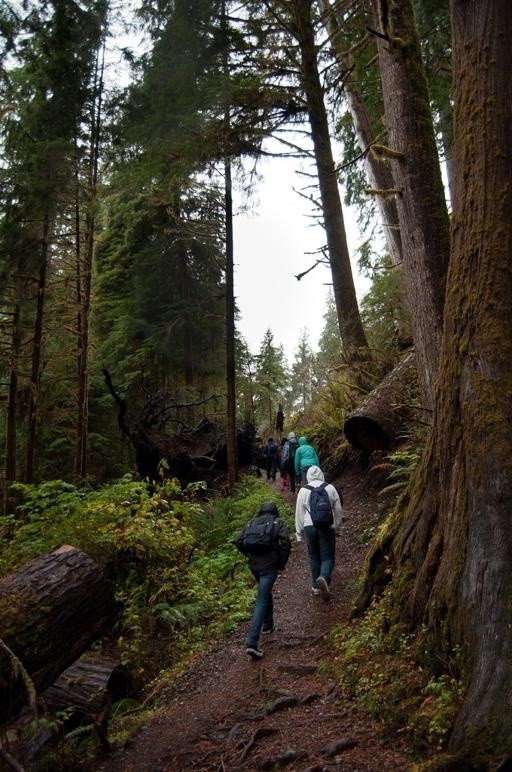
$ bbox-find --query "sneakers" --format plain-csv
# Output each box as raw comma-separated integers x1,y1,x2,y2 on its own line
312,576,330,601
261,624,275,634
246,648,264,658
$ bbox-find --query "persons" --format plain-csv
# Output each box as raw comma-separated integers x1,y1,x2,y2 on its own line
295,465,342,601
256,432,320,494
236,502,291,658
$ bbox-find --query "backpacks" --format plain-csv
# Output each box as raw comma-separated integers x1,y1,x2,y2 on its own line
268,444,277,459
287,440,300,460
243,516,277,555
303,482,333,529
